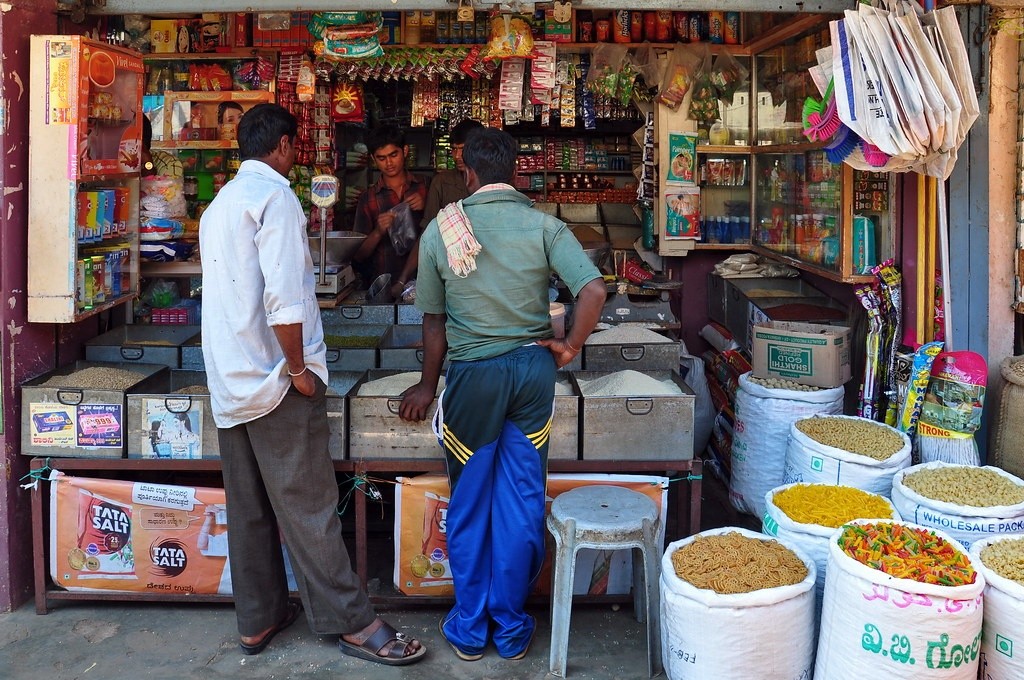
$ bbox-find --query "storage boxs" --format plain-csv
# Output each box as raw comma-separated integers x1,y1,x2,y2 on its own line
18,358,169,461
571,367,696,461
126,368,222,460
375,325,452,370
549,369,582,460
344,366,446,459
706,270,803,327
751,320,853,388
320,322,398,370
324,371,366,460
745,297,861,362
180,332,205,370
319,289,401,324
83,324,203,369
396,295,425,324
581,328,681,377
558,346,583,370
725,278,828,349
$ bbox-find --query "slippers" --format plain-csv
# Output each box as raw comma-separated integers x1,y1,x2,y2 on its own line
337,622,427,666
239,597,302,655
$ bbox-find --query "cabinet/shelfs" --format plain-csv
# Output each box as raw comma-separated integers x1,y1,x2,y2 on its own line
24,9,901,323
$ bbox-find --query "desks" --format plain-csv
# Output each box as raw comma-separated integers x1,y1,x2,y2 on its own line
28,453,705,616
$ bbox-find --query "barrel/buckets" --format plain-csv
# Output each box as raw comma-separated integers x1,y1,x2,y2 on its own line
549,302,565,338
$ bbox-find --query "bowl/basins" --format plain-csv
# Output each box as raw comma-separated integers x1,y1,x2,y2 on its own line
306,231,368,265
583,245,608,271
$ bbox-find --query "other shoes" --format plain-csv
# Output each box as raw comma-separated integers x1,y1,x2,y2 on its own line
439,616,483,661
506,616,537,659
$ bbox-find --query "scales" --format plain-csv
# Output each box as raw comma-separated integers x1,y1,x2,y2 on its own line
307,175,369,300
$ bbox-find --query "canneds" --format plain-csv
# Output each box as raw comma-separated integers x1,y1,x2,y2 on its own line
579,8,739,44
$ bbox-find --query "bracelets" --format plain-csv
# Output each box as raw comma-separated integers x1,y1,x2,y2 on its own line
287,366,307,376
566,337,582,352
397,280,405,286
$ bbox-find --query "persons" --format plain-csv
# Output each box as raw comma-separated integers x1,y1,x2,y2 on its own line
388,120,485,296
196,503,300,595
148,418,169,452
351,123,432,286
198,103,429,667
216,101,245,140
169,413,197,460
396,125,608,662
670,194,693,215
673,154,691,177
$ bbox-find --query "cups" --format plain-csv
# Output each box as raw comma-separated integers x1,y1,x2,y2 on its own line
365,273,394,305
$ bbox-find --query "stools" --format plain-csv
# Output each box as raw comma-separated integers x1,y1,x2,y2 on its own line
545,484,665,680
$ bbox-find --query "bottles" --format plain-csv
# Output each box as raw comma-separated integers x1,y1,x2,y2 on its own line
757,208,823,256
700,215,750,244
